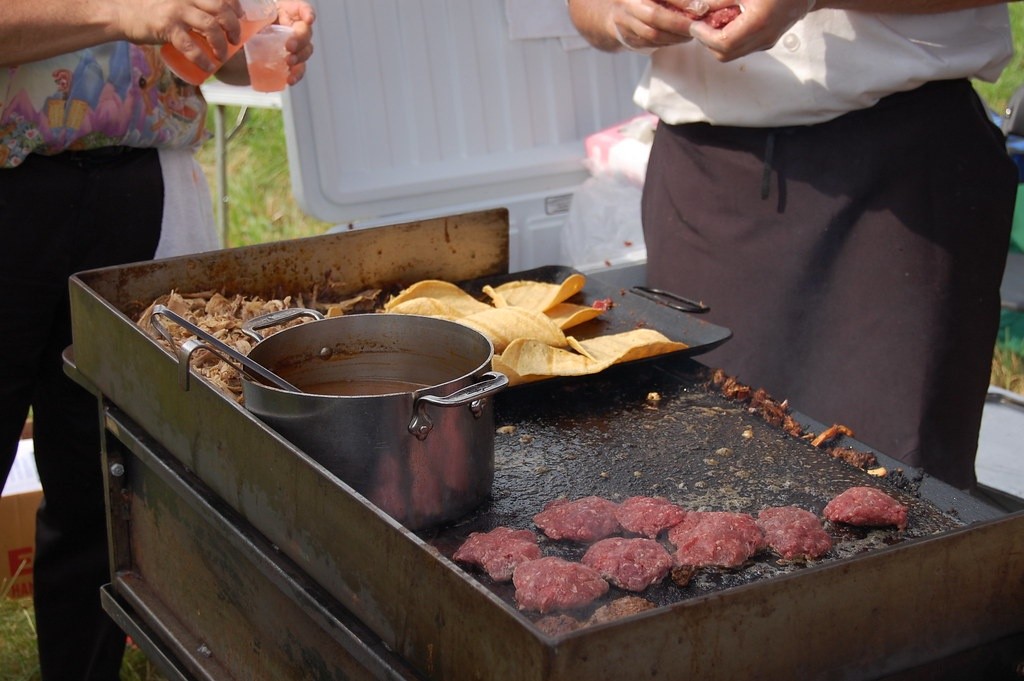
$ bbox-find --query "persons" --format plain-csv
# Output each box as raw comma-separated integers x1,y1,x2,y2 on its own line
564,0,1021,485
0,0,312,681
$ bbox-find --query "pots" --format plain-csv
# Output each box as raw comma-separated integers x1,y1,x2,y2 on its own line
236,300,511,536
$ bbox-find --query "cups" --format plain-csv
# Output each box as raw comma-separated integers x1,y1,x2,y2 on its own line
243,23,296,92
159,0,276,84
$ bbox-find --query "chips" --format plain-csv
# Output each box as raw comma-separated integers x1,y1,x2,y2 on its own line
381,272,689,387
